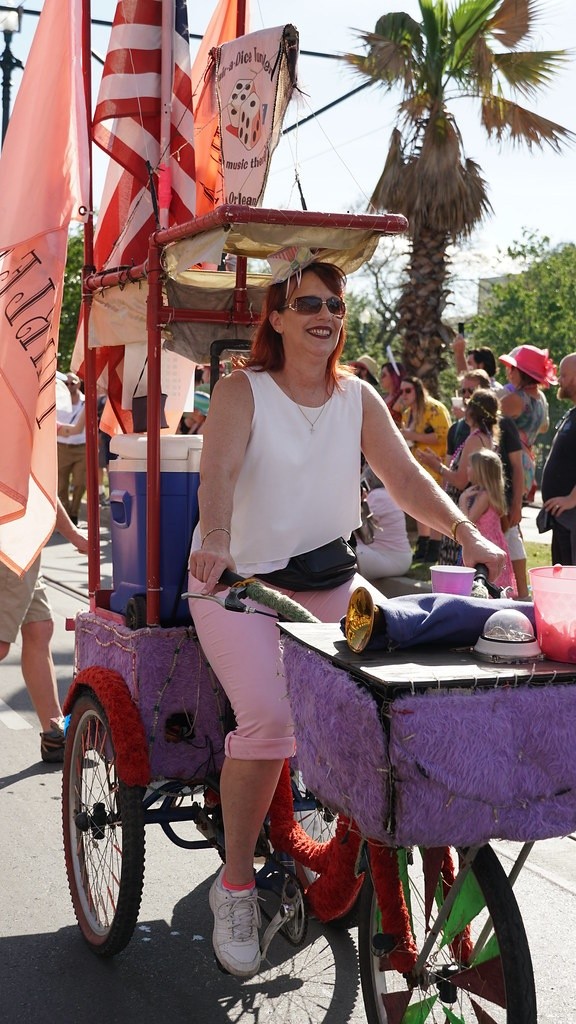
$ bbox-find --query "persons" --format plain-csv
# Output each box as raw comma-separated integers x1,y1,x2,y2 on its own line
541,351,576,566
187,259,509,978
176,356,243,436
56,372,113,526
347,335,552,601
0,498,89,763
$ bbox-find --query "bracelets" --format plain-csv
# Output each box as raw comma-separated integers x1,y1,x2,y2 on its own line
450,519,476,543
201,527,231,548
440,466,445,474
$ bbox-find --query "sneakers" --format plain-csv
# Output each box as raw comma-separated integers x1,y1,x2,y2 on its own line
209,863,268,976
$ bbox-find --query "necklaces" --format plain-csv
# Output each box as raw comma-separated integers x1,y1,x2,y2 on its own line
445,428,480,491
286,379,326,434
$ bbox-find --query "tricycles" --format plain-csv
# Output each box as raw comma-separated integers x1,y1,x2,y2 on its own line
62,201,576,1024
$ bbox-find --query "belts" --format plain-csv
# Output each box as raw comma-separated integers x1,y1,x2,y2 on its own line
57,442,86,449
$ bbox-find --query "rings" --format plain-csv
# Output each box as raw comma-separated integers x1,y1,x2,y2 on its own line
501,565,506,570
556,504,559,508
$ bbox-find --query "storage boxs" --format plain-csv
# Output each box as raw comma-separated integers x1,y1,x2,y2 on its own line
110,433,207,627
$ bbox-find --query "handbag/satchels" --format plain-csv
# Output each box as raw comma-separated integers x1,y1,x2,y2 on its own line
254,534,359,592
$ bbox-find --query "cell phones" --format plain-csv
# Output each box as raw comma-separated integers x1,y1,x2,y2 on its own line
458,322,465,338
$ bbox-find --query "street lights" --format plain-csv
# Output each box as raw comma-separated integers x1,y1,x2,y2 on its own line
0,0,24,150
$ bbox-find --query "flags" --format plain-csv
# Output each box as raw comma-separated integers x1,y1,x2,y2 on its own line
71,0,197,436
0,0,89,575
189,0,249,271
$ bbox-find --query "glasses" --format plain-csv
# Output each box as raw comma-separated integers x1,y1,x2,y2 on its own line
459,386,474,396
379,372,392,379
399,386,417,395
65,380,80,386
275,294,346,315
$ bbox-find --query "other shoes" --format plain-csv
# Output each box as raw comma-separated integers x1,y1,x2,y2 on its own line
411,541,428,563
415,560,438,575
99,493,111,506
40,724,94,763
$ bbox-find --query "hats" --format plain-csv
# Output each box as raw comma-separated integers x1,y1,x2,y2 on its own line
498,344,559,388
348,355,381,385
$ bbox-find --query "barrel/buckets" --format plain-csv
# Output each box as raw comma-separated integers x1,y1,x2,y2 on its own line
528,563,576,663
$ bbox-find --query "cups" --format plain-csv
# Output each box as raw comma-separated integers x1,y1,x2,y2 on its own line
429,565,477,597
451,397,463,409
